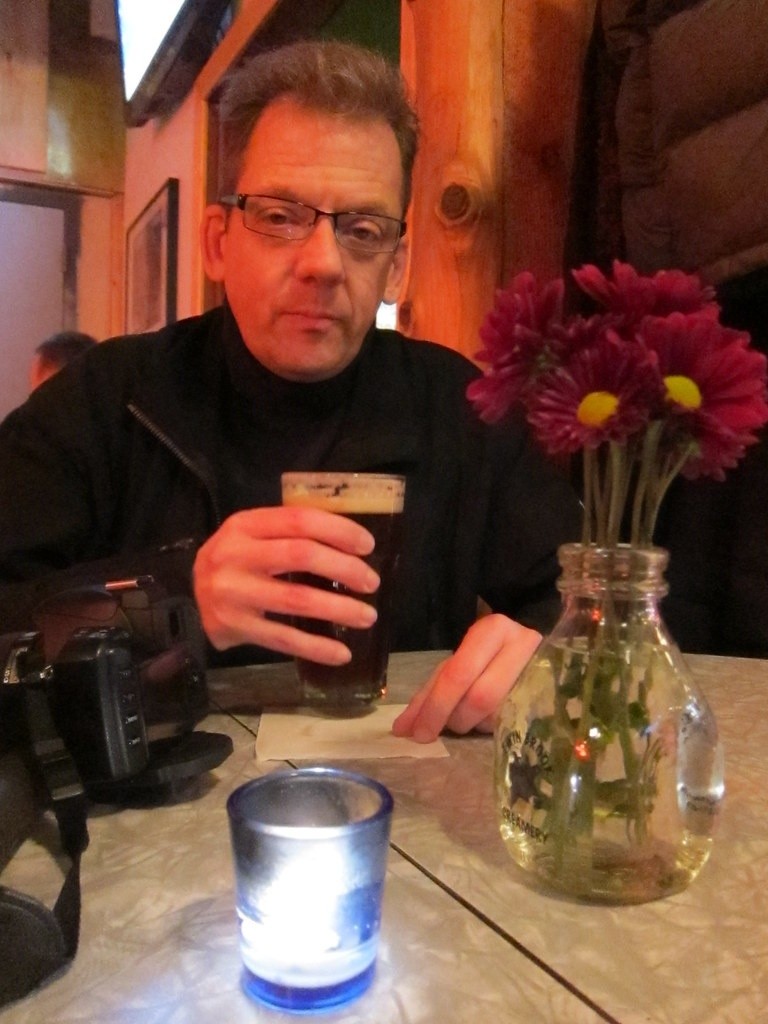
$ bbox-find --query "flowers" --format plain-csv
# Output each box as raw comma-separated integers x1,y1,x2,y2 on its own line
466,257,768,876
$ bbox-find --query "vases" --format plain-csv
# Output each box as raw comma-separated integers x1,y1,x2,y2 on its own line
488,542,724,905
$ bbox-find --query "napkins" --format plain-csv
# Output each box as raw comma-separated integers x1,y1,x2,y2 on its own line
254,704,450,761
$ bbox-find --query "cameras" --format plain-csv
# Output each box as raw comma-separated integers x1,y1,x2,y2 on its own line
0,627,150,794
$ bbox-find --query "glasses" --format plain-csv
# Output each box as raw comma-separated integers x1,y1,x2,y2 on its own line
218,193,407,253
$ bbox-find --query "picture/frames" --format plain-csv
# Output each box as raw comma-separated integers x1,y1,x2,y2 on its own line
124,177,179,336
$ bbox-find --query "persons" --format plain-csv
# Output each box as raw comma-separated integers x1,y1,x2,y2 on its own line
29,327,100,397
0,41,604,743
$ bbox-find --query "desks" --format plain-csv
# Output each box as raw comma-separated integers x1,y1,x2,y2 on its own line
0,636,768,1024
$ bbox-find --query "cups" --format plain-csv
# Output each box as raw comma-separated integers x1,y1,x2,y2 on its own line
225,765,393,1013
281,471,407,717
